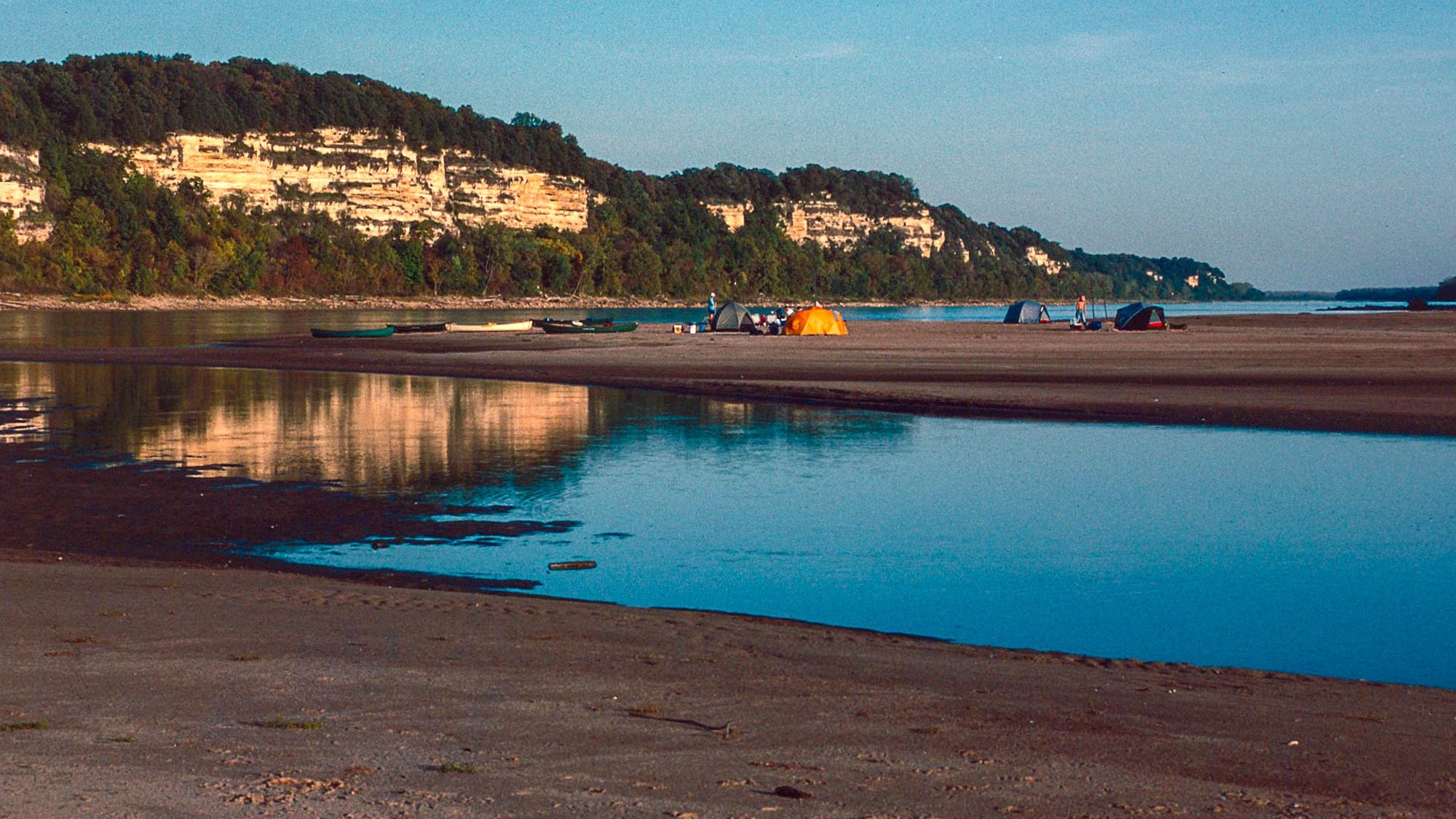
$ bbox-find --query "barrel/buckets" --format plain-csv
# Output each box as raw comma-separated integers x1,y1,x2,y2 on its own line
689,324,696,334
699,324,706,332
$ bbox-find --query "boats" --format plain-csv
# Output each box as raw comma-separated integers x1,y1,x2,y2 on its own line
385,319,455,333
528,317,617,328
310,326,394,337
444,321,533,332
542,319,639,334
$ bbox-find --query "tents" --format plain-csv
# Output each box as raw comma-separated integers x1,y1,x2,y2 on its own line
1002,300,1052,324
711,302,755,333
1114,302,1168,331
782,306,849,337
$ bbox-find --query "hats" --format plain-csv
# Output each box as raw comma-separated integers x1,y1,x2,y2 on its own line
1081,295,1086,301
710,292,716,297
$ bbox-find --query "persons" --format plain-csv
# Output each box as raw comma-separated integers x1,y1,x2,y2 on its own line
1074,295,1088,329
708,293,718,321
767,304,804,327
